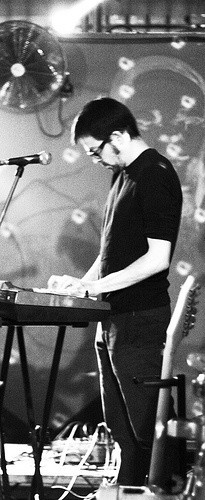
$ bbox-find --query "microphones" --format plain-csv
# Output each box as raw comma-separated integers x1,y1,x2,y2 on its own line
0,152,52,166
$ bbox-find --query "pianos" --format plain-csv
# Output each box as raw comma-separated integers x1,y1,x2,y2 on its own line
5,281,113,326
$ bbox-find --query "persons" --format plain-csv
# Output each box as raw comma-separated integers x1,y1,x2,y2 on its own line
49,96,183,500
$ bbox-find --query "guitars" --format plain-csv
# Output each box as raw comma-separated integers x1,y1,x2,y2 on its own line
144,274,205,487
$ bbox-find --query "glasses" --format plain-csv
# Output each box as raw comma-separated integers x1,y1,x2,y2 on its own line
87,131,123,159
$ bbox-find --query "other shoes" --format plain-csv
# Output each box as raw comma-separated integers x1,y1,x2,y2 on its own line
84,476,144,500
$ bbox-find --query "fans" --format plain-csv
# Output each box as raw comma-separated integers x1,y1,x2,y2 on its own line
0,20,73,115
